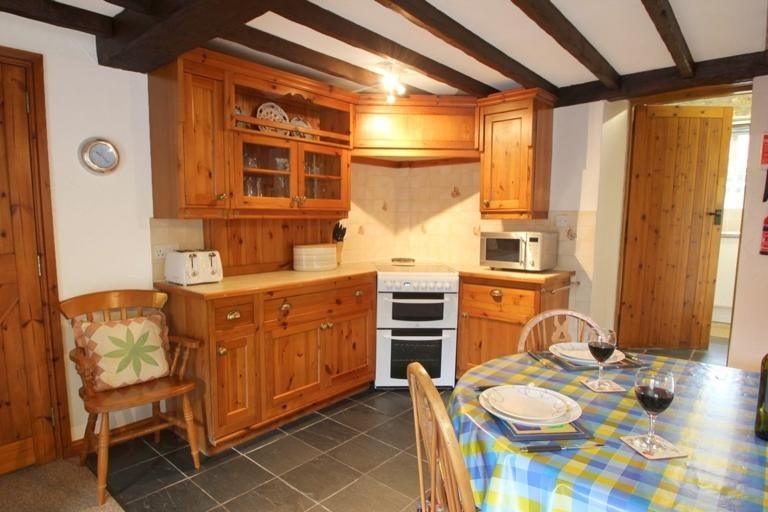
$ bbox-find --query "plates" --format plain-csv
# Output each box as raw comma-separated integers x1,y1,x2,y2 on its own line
549,342,625,365
256,102,290,136
478,386,582,427
290,117,312,138
559,346,594,359
489,388,568,420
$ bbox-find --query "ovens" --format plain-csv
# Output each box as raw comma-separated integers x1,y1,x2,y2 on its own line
376,272,459,391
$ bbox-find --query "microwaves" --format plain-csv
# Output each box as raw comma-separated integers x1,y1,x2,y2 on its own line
479,230,558,271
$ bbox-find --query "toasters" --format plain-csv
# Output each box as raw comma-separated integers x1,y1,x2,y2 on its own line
165,248,223,286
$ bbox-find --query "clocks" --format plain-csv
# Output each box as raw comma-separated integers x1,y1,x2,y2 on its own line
81,139,120,174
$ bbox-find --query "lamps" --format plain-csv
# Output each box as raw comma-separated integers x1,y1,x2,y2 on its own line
378,58,406,105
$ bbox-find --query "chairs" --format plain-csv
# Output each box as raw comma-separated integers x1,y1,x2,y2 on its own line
58,289,201,505
516,308,606,355
407,362,475,512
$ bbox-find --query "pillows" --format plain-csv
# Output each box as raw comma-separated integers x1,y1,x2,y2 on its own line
73,312,173,393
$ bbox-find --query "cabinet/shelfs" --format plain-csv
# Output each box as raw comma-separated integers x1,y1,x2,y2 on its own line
230,73,353,220
456,270,576,384
206,293,262,442
478,99,536,221
260,276,376,421
177,57,230,220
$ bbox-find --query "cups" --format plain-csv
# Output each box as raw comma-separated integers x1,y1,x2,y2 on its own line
587,327,616,387
633,366,675,451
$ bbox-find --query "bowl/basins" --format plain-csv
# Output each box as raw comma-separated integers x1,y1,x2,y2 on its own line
293,243,335,270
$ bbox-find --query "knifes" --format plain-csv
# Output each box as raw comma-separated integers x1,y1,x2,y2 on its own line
520,443,604,453
